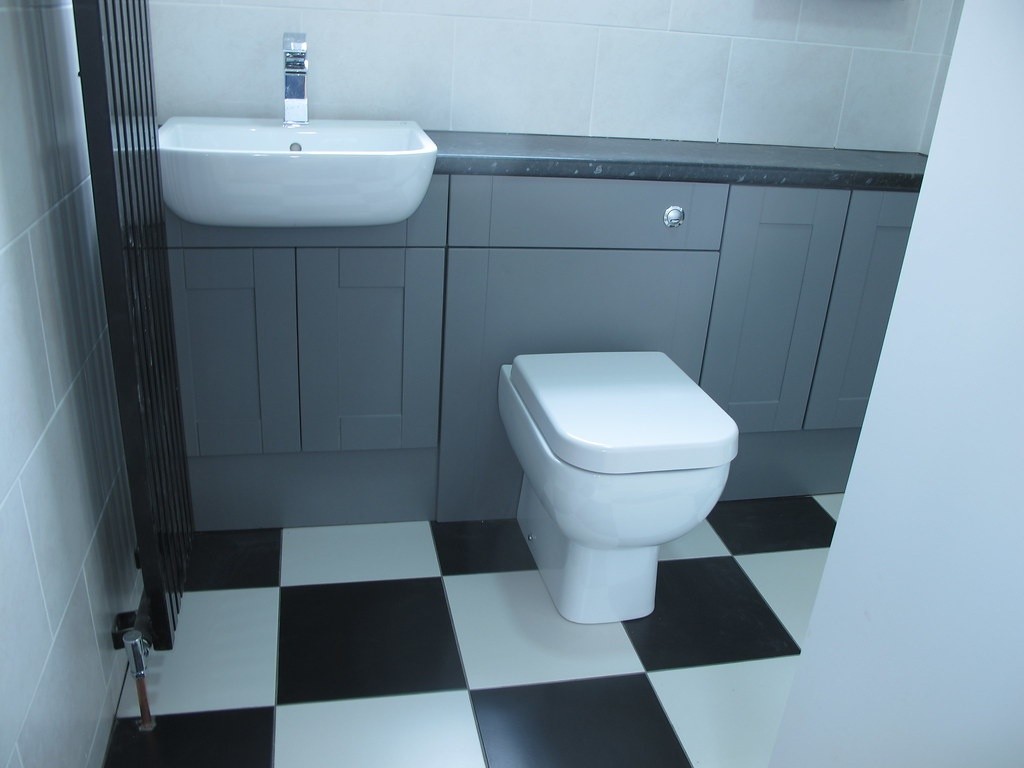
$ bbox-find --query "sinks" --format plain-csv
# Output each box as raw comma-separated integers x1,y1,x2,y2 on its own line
157,116,438,229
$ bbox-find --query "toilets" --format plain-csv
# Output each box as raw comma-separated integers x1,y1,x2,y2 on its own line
497,350,739,624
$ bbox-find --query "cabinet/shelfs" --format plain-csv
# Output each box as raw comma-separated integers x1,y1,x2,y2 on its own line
124,175,920,456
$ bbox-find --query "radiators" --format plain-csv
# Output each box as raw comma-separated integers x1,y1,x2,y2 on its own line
71,0,196,731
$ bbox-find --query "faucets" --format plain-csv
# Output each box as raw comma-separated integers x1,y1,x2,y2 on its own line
282,32,310,128
